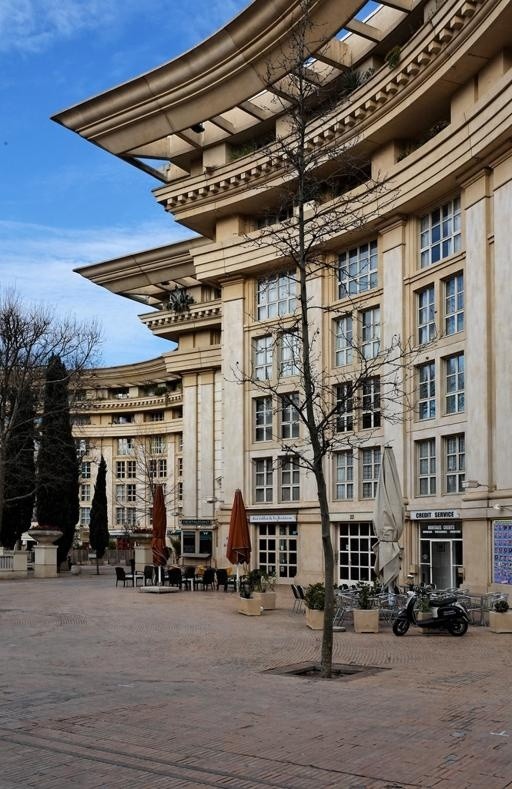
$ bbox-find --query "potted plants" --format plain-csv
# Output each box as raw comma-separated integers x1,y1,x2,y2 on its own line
237,567,277,615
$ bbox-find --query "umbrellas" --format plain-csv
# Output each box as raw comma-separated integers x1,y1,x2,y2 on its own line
226,487,251,579
149,483,169,585
374,445,406,596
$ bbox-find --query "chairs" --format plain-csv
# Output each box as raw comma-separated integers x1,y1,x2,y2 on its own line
115,565,237,593
290,582,509,633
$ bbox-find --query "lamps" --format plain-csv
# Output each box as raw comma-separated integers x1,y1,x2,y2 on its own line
493,503,504,512
462,480,488,488
206,497,224,503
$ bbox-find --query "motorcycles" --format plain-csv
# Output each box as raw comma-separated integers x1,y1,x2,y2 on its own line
392,583,471,638
